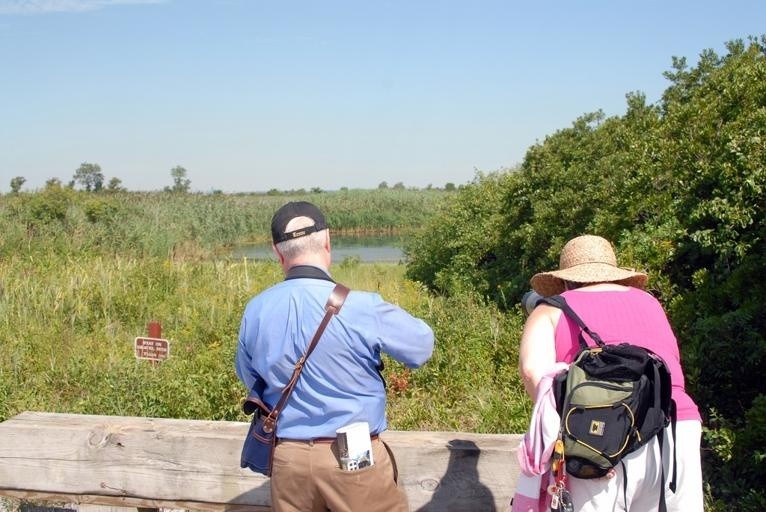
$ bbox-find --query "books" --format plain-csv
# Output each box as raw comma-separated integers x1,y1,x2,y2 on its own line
335,422,374,473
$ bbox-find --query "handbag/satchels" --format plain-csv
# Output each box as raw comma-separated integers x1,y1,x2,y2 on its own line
240,379,278,478
553,342,672,478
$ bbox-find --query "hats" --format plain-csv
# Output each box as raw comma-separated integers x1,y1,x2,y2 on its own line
271,202,328,243
530,235,648,297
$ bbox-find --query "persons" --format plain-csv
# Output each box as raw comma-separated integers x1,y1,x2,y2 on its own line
517,235,707,511
233,202,438,511
